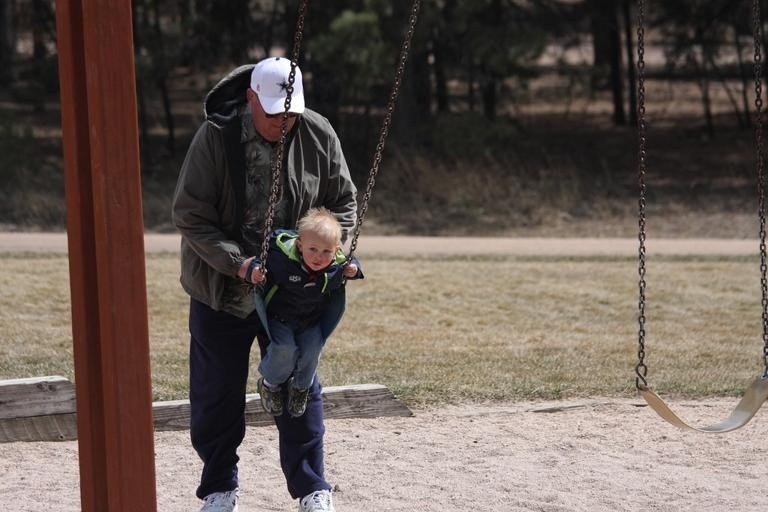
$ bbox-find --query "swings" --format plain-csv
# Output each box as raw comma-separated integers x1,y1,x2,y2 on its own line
637,1,768,433
254,0,420,343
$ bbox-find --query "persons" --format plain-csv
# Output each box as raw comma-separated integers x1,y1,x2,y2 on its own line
172,55,365,512
253,208,365,418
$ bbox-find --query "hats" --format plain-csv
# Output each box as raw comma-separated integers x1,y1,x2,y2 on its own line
250,56,305,116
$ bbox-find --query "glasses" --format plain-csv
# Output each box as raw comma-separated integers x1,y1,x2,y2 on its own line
252,90,300,119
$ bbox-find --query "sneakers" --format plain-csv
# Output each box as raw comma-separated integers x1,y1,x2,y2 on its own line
256,377,311,419
195,487,238,512
296,489,334,512
